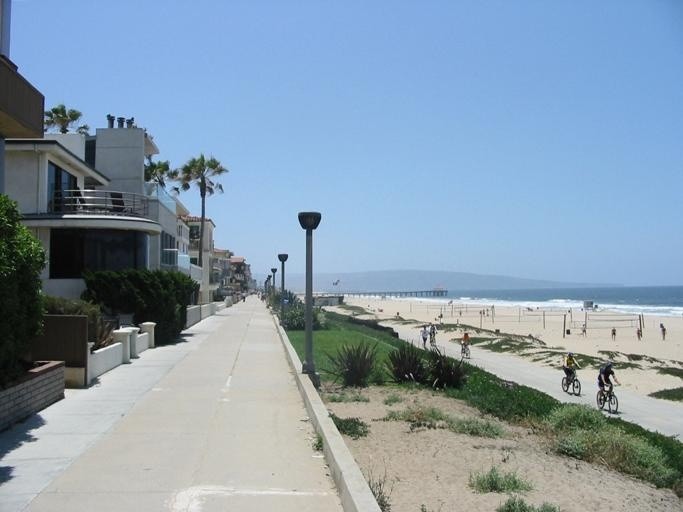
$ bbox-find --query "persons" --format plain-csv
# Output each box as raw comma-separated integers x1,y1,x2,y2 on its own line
662,326,666,341
461,332,473,353
596,361,622,405
419,326,432,349
612,327,617,340
637,327,642,340
562,352,582,386
582,324,586,337
428,324,439,342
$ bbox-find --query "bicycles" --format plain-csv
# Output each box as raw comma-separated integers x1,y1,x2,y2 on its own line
430,334,435,346
461,343,470,358
561,377,581,395
596,385,618,413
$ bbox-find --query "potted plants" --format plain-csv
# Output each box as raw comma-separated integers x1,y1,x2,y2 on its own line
107,113,135,128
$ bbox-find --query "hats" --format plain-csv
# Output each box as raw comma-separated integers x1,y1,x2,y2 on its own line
607,363,613,368
569,353,573,356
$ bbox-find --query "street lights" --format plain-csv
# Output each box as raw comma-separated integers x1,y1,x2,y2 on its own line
278,254,288,319
299,213,321,373
264,269,277,299
